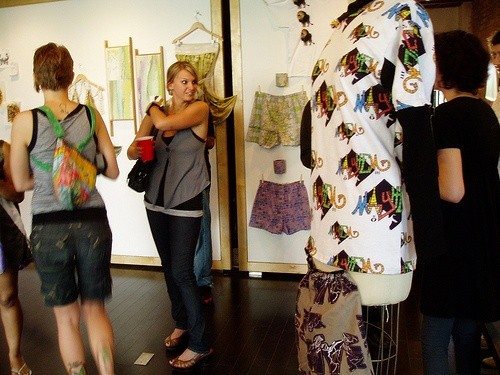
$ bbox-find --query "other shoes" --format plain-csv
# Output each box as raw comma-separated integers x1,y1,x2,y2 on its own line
480,335,489,349
200,285,212,303
482,357,496,369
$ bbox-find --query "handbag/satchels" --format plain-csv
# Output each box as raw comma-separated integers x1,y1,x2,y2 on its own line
52,138,97,210
128,158,153,192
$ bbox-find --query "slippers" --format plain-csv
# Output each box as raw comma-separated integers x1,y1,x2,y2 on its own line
10,362,32,375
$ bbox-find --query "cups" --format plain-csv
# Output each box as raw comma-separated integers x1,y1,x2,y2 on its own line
135,136,153,161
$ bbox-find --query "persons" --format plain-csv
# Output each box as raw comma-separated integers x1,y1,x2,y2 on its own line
423,30,500,375
10,42,119,375
0,140,32,375
127,61,215,372
489,32,500,70
306,0,435,305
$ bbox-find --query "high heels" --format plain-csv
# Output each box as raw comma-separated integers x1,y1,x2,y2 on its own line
163,327,185,348
169,345,214,369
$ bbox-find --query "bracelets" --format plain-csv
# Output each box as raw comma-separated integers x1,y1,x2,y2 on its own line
145,102,160,116
